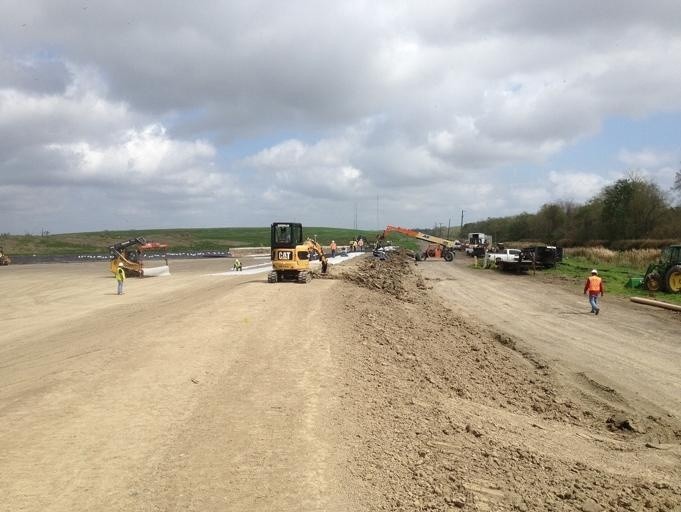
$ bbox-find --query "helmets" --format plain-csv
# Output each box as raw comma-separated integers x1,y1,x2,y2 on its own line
118,262,124,267
591,269,598,274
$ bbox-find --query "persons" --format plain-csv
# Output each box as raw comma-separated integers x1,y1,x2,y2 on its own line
232,258,242,271
583,268,604,316
358,238,364,252
329,240,337,258
115,262,125,296
351,239,358,252
423,247,430,261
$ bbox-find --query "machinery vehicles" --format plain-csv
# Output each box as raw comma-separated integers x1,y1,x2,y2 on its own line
267,221,328,284
109,236,146,278
625,244,681,294
384,225,456,262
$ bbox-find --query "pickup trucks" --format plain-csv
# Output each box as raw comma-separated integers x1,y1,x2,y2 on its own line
488,248,535,265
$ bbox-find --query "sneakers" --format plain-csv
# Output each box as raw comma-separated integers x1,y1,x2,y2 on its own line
590,308,600,315
